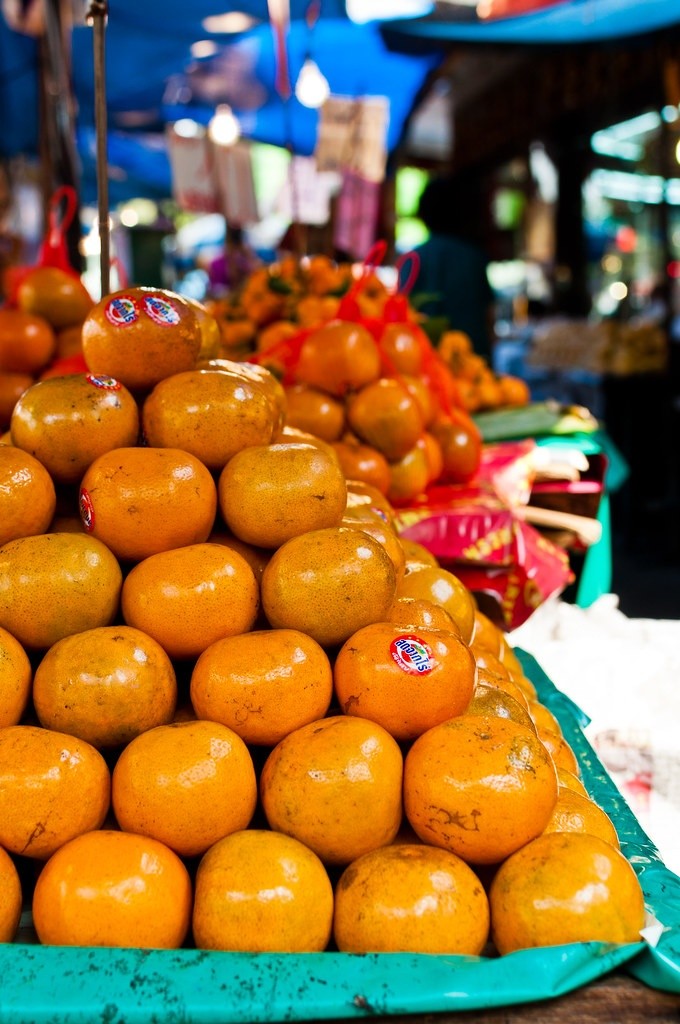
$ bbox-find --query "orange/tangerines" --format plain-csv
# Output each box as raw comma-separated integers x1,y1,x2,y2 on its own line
0,255,660,958
